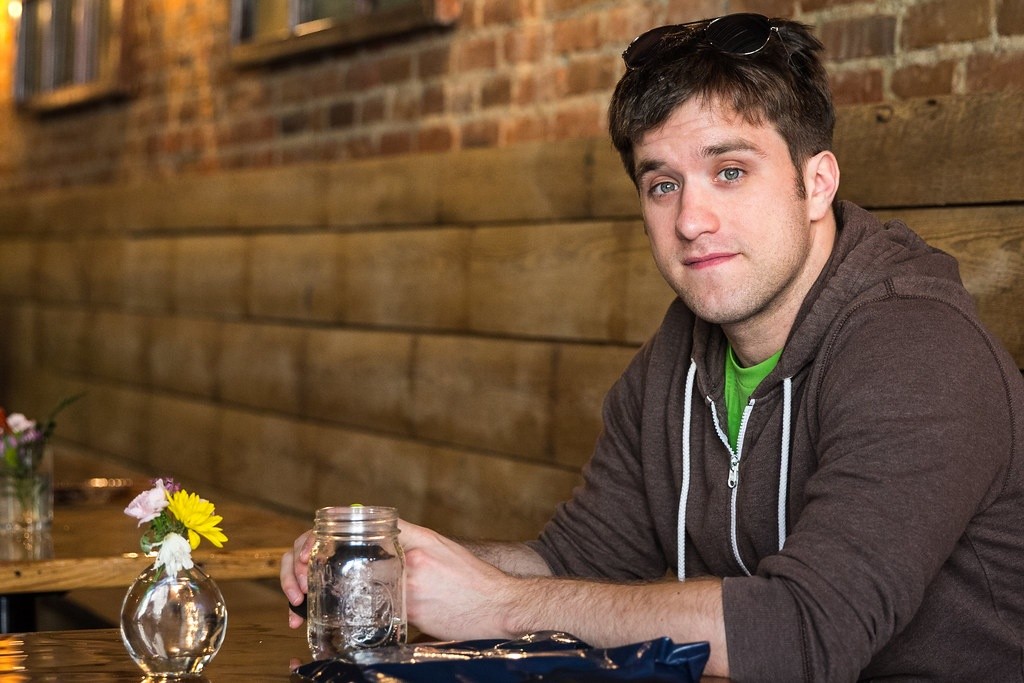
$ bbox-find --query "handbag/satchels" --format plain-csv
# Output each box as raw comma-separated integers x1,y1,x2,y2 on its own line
289,628,711,683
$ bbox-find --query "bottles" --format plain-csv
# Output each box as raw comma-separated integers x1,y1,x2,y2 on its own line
306,505,409,662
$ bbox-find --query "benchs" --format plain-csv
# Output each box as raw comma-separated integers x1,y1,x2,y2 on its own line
65,579,305,626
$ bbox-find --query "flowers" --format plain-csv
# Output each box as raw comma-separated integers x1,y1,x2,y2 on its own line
0,389,93,511
124,478,229,598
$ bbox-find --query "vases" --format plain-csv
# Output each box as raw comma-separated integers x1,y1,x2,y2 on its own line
0,442,55,565
120,563,229,683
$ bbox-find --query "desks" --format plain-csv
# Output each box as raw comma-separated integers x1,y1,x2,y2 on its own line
0,623,740,683
0,452,318,633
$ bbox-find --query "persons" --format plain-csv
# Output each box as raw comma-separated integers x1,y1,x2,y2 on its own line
278,17,1024,683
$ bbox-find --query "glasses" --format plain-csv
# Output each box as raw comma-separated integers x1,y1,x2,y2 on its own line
622,12,795,70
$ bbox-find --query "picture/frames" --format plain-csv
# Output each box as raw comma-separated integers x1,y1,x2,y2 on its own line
228,0,459,64
16,0,142,117
